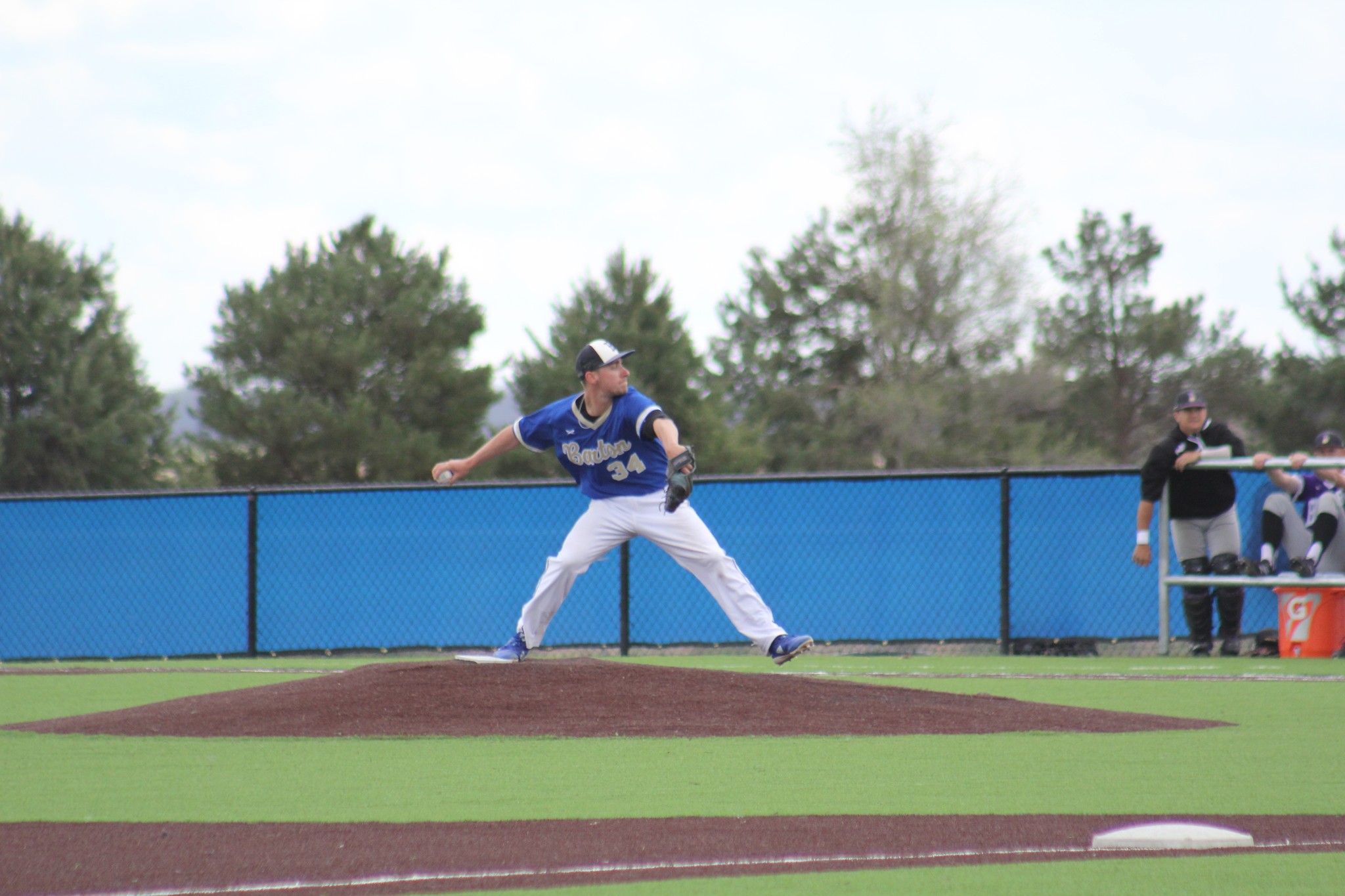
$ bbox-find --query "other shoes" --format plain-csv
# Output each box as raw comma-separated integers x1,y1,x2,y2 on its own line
1288,557,1316,577
1190,640,1213,657
1220,636,1240,656
1235,557,1275,577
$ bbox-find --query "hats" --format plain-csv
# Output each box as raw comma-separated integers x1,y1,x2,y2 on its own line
1313,430,1345,449
1172,390,1206,411
575,338,636,382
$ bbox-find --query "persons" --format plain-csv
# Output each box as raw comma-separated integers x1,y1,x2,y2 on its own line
1131,390,1246,656
1245,432,1345,578
432,340,814,666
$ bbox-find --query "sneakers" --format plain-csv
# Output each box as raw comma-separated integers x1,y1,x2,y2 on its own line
494,632,531,663
768,634,814,666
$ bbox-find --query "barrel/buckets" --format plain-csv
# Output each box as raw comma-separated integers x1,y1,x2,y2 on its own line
1272,570,1345,658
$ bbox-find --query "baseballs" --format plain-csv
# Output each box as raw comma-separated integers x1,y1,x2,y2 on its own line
435,470,453,485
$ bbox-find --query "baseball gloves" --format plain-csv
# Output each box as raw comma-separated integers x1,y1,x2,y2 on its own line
664,444,699,514
